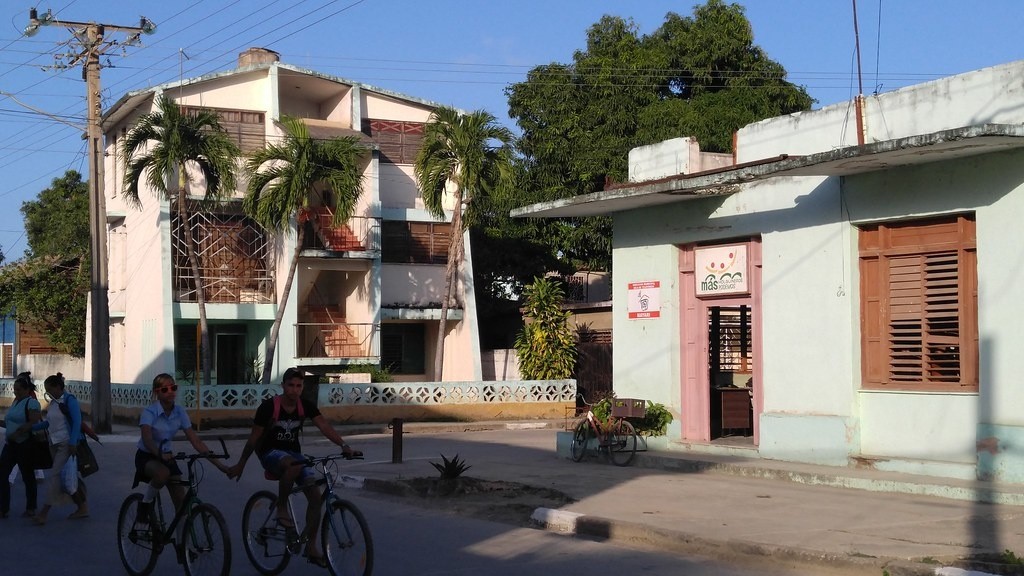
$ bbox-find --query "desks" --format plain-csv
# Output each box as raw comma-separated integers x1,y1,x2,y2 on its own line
711,387,750,437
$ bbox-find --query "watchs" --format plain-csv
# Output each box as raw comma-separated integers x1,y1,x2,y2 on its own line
341,443,346,447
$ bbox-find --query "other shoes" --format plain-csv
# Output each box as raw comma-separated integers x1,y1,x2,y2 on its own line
22,509,36,517
67,511,89,520
176,543,198,565
136,502,157,524
32,517,46,526
0,512,9,518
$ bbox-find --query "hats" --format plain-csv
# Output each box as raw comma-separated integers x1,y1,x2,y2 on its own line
283,368,304,383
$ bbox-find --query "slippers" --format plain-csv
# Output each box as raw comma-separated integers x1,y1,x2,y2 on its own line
277,516,295,528
304,549,334,568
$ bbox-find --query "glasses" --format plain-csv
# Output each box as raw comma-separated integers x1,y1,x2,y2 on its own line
155,385,178,393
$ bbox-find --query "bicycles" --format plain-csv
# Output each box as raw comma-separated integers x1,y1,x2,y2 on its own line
116,436,232,576
570,392,638,467
242,450,376,576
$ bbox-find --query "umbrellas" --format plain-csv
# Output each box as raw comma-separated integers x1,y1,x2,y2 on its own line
44,390,104,447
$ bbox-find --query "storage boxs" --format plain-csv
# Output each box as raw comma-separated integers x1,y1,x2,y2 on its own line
611,398,645,418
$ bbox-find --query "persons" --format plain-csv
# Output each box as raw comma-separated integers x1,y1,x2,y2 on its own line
0,378,41,518
229,367,352,568
134,373,246,564
8,371,45,486
32,372,91,525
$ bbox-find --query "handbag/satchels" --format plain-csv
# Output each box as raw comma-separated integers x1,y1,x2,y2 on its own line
61,455,78,495
25,397,54,469
69,439,99,478
